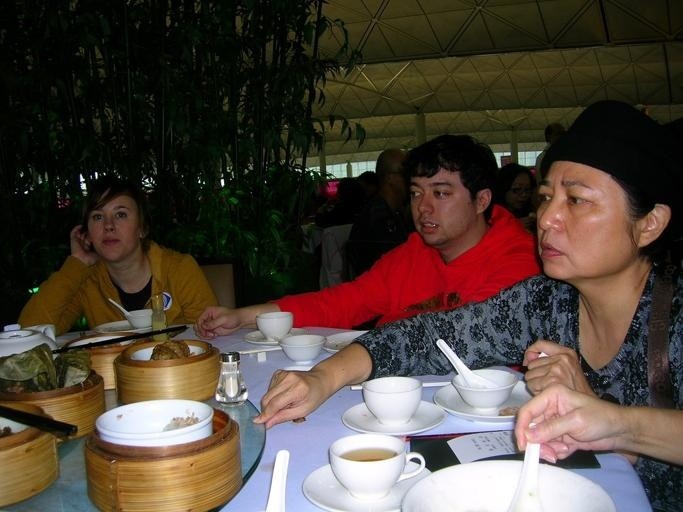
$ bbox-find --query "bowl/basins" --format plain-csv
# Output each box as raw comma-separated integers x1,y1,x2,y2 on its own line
360,374,422,427
450,368,519,413
255,310,293,341
278,333,326,365
0,322,57,362
123,308,152,329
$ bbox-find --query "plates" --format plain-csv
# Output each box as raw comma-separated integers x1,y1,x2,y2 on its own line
339,398,444,436
320,330,371,355
432,377,538,423
92,319,151,333
400,459,620,512
243,326,309,345
302,462,432,510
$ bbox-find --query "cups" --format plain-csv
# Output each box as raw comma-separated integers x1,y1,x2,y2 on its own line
327,431,425,499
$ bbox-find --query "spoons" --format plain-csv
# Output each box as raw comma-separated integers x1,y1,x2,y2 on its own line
507,424,545,512
433,336,497,389
265,449,289,511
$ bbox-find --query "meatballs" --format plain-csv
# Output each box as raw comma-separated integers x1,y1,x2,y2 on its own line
151,341,191,360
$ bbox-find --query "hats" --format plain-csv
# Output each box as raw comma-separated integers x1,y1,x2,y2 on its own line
540,100,682,204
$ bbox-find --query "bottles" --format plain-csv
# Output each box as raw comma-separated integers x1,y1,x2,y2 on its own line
213,351,249,408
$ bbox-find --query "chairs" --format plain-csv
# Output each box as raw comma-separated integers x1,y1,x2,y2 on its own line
319,221,355,292
194,255,244,311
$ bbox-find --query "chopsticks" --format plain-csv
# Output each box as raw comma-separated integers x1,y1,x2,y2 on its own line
0,405,78,443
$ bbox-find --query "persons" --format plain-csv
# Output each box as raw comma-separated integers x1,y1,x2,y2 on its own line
315,124,565,279
253,99,683,512
515,382,683,466
18,176,219,338
197,135,541,371
